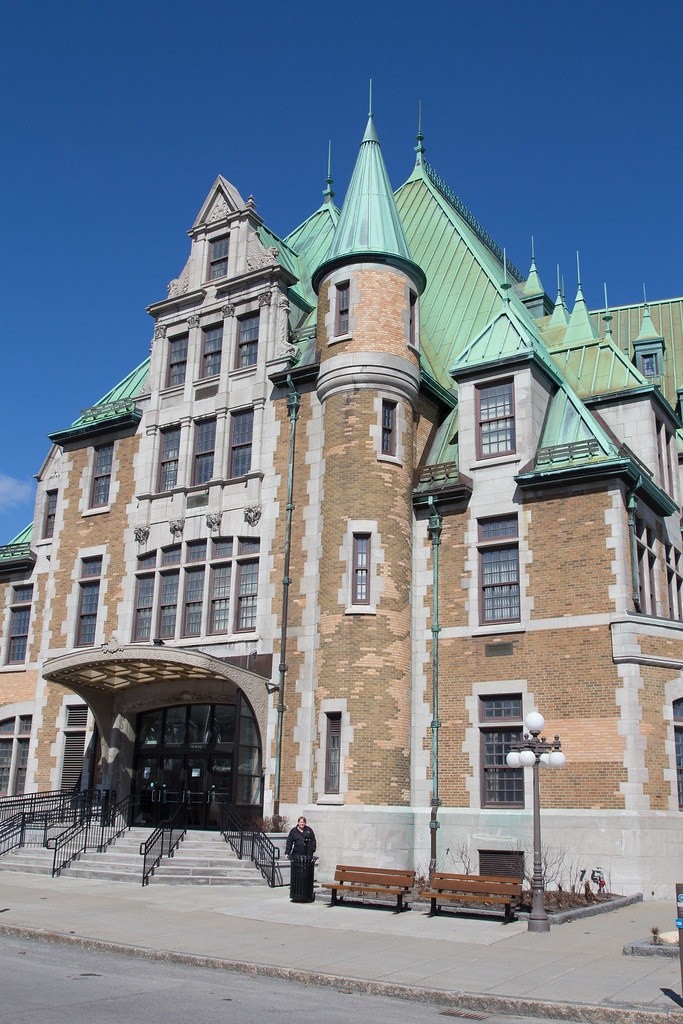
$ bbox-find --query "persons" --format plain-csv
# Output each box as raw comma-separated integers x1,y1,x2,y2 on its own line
285,816,317,899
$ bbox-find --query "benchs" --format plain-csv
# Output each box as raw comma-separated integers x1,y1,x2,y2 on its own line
421,873,524,924
321,865,416,915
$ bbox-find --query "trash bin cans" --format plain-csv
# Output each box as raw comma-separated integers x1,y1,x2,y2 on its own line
288,855,318,903
